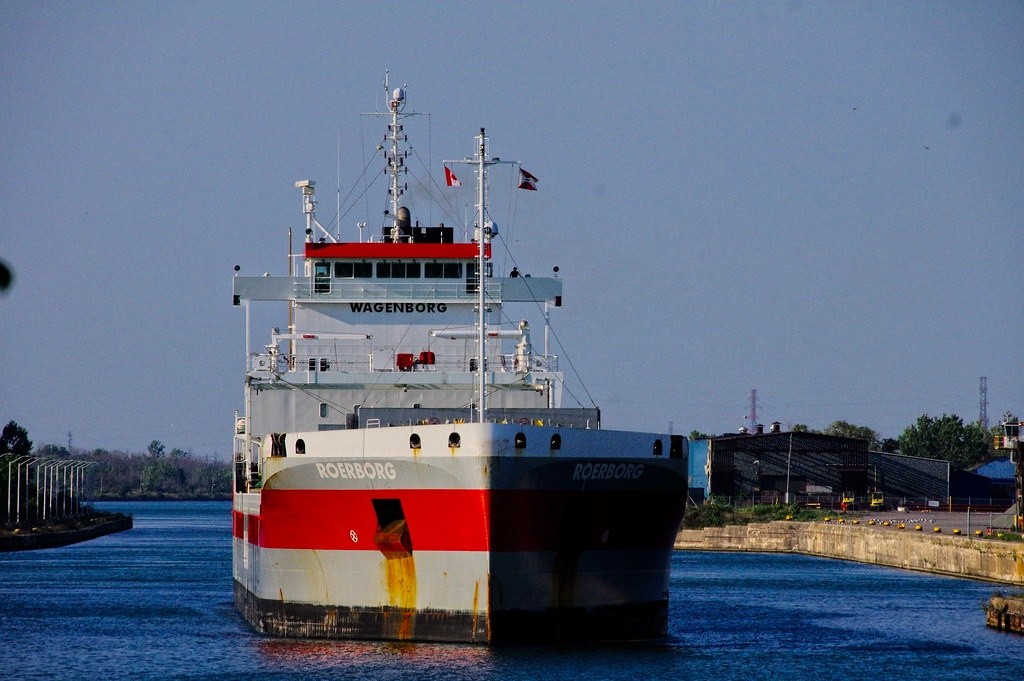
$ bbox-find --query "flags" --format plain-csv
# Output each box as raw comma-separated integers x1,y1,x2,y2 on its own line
518,168,538,191
444,166,462,187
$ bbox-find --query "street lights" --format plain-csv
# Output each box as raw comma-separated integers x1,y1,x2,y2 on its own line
0,452,98,529
784,432,793,503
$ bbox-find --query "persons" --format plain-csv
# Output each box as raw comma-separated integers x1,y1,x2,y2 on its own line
510,266,521,277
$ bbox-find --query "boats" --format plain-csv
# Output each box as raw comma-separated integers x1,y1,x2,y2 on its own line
231,69,685,655
